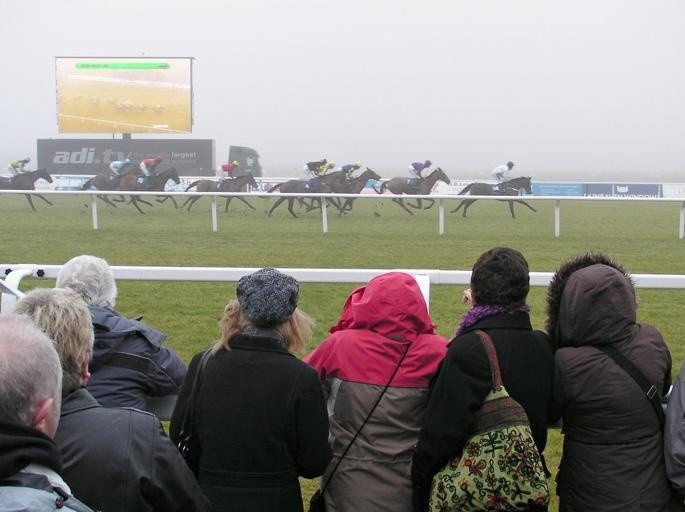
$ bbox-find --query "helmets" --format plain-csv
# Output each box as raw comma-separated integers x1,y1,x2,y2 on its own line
155,157,163,162
23,156,31,162
125,158,132,164
329,162,335,166
507,162,513,166
320,157,328,163
355,161,362,167
233,160,240,166
425,160,432,165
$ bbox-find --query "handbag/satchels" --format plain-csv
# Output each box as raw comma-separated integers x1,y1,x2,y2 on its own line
309,488,326,512
428,385,550,511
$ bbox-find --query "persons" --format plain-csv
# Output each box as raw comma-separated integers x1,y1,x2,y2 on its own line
7,156,30,184
10,288,214,512
408,244,556,510
299,272,452,511
491,161,515,191
0,310,95,512
318,161,336,175
542,248,674,512
303,157,328,189
215,159,241,190
408,159,432,182
660,355,685,511
109,158,130,182
166,266,334,512
55,253,188,413
139,155,163,177
341,161,362,181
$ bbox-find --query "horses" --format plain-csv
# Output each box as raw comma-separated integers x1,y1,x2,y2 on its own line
450,176,538,218
0,168,54,211
180,173,259,212
78,167,180,216
371,168,451,217
256,167,382,219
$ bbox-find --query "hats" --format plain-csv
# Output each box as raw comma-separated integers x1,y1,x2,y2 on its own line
235,265,300,326
